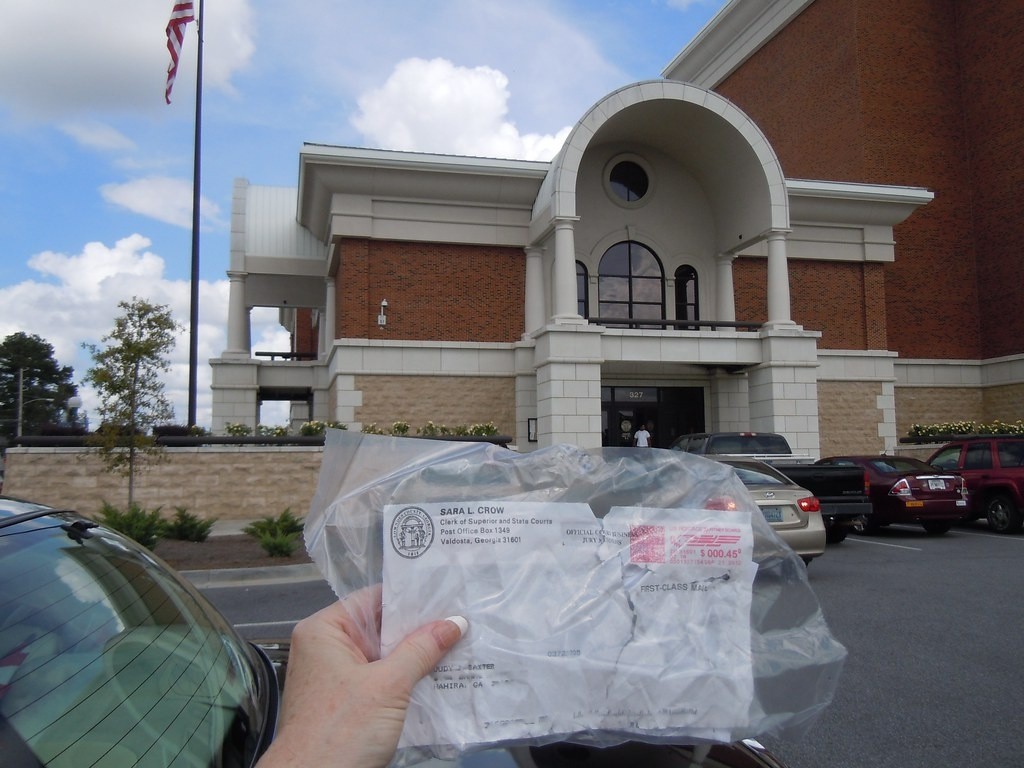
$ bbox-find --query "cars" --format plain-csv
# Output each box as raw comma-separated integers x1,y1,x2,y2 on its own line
0,495,296,768
811,455,969,537
923,435,1024,539
661,452,829,576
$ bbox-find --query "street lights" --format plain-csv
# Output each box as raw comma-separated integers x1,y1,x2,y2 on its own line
19,396,56,449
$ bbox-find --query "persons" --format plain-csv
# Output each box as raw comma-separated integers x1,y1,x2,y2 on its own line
632,424,652,448
245,585,469,768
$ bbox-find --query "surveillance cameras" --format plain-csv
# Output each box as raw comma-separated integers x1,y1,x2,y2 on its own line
382,301,388,307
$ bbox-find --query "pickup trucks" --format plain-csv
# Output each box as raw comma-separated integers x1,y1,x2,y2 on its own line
668,432,873,549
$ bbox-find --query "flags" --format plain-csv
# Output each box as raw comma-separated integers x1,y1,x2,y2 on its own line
163,1,194,105
